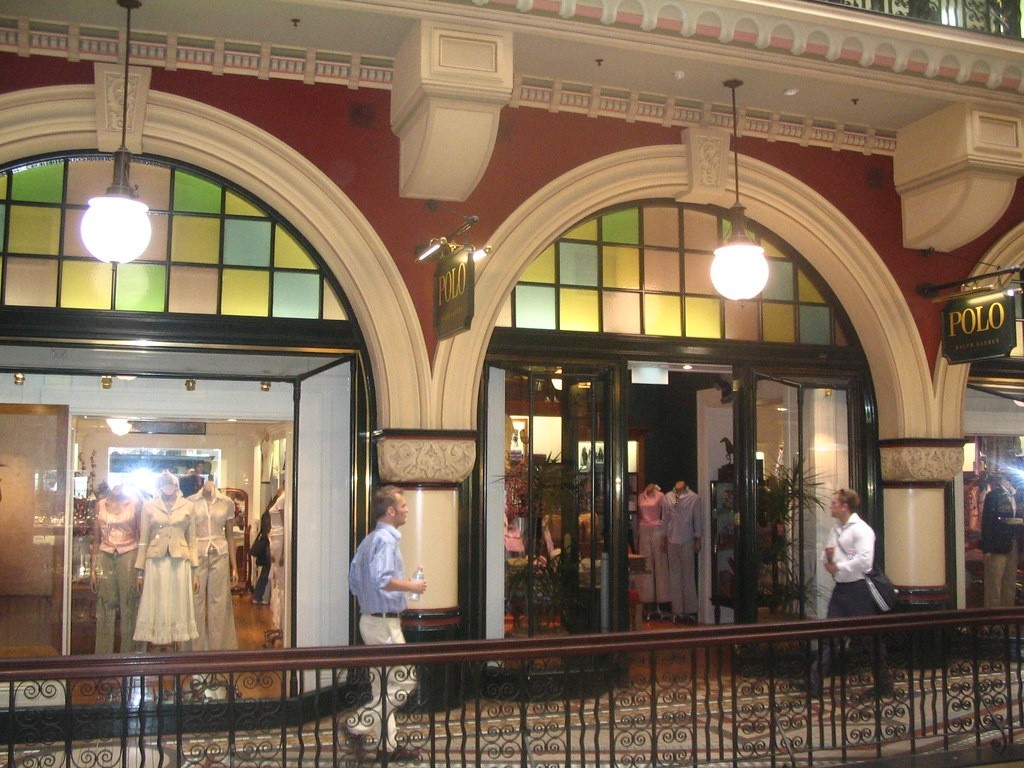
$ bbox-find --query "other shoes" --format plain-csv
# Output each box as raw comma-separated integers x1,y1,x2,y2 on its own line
341,724,377,762
377,746,426,762
860,687,894,699
252,599,268,605
788,680,821,701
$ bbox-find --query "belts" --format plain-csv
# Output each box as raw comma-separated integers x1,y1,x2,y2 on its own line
370,612,401,618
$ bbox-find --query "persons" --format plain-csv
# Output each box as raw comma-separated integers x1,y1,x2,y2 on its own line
265,490,286,643
660,481,704,624
133,485,201,652
251,495,277,604
260,579,272,606
338,484,426,764
790,487,898,700
978,478,1022,608
191,460,207,487
636,482,672,618
184,480,239,651
90,484,142,654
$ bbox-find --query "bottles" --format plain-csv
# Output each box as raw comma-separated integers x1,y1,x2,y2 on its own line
409,566,424,601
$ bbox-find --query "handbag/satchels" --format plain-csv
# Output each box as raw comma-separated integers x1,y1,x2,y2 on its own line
249,529,271,560
864,565,899,612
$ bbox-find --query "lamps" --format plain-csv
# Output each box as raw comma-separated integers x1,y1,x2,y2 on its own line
710,79,770,308
80,0,152,270
